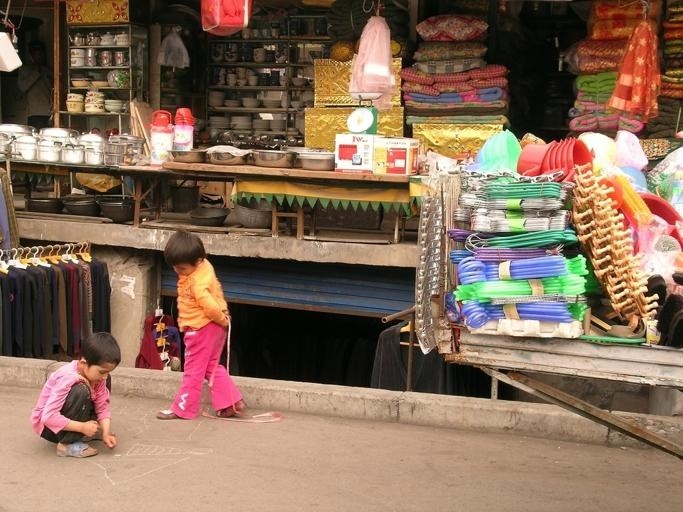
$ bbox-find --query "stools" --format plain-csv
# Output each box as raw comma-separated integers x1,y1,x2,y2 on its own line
271,196,317,240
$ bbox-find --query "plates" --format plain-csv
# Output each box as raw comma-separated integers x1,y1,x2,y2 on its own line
209,90,280,108
70,79,108,87
208,116,286,133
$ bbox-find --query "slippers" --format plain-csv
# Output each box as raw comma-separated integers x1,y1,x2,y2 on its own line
56,441,97,458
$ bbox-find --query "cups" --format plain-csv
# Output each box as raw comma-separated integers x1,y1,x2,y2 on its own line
241,27,281,41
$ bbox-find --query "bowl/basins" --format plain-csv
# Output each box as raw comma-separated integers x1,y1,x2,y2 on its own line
252,48,266,63
27,195,134,222
290,78,315,108
167,145,335,170
226,68,257,86
626,192,683,259
64,91,124,114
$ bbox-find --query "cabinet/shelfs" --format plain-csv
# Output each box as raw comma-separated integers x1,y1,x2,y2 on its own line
149,21,190,125
64,25,150,137
203,16,331,148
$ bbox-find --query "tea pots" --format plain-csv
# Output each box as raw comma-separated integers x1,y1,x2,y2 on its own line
68,30,129,47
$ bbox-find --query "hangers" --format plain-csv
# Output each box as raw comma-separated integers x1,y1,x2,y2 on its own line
396,306,427,348
445,176,589,329
0,240,106,279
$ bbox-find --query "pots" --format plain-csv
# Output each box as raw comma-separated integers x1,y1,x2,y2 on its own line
0,121,148,167
188,206,230,226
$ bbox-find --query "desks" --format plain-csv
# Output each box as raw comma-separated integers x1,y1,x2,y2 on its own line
0,153,188,228
230,179,413,243
165,161,421,246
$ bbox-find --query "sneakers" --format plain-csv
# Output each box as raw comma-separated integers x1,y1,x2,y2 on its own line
217,401,246,419
157,410,179,421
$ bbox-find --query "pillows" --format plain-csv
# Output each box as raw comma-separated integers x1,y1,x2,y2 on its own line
413,38,490,62
415,14,490,41
401,61,510,95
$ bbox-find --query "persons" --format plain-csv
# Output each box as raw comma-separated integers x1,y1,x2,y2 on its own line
29,331,121,459
155,230,246,420
